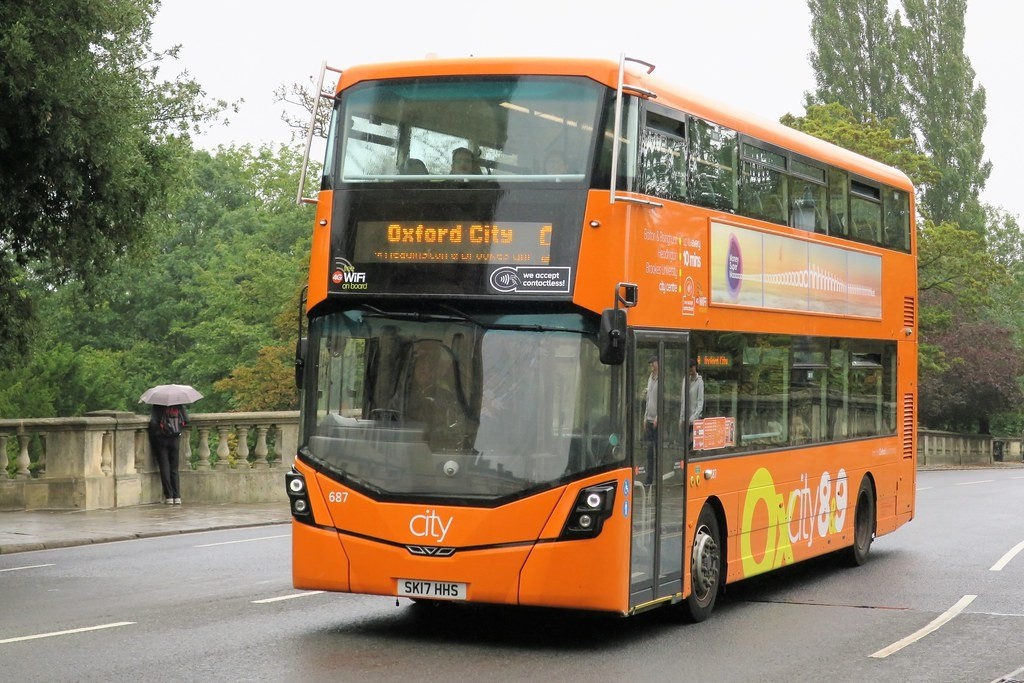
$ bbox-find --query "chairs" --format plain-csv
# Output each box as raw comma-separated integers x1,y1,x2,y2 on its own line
644,161,904,252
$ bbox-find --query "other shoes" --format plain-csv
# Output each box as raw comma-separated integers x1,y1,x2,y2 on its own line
175,498,181,504
165,498,173,505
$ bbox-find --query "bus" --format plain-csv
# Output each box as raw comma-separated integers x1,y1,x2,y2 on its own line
284,53,922,622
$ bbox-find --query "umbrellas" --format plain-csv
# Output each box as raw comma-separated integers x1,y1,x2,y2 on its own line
139,384,204,406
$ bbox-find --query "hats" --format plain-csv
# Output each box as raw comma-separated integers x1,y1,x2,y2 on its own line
649,356,659,363
685,359,698,366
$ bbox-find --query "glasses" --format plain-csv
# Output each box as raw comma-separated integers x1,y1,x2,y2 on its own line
453,158,472,164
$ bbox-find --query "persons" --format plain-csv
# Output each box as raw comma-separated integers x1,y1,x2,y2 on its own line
148,401,190,505
447,147,477,179
677,357,705,459
401,361,454,422
545,148,571,179
643,355,666,490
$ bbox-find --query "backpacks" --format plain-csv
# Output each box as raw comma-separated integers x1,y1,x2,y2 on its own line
160,407,185,440
